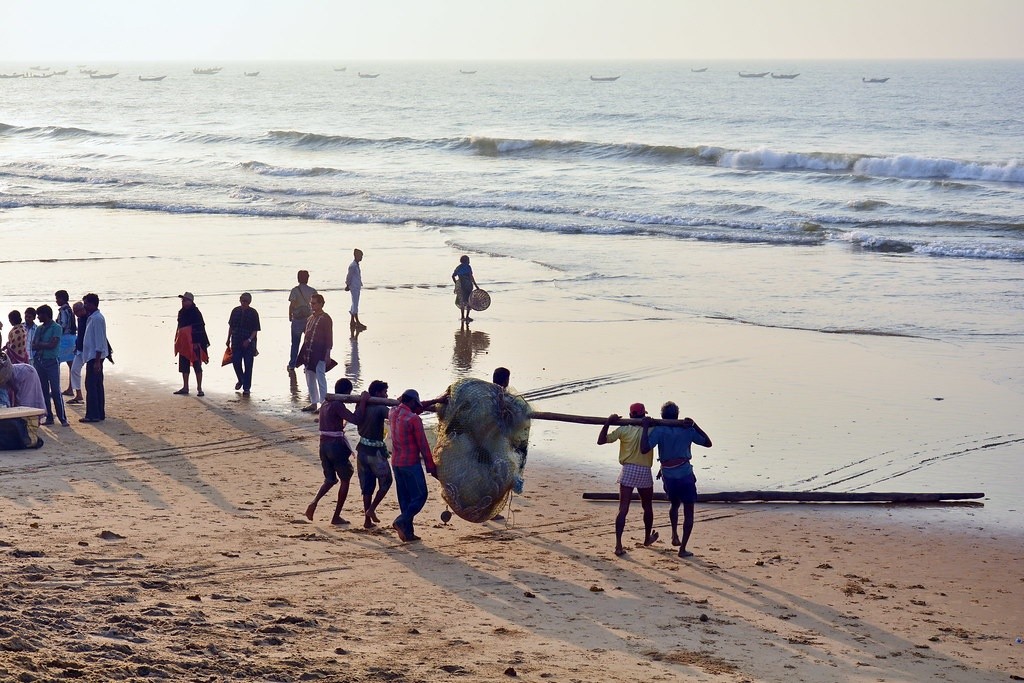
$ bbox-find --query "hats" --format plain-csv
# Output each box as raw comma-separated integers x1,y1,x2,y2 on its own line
178,292,194,300
240,293,252,304
73,302,84,313
404,389,424,407
629,403,648,416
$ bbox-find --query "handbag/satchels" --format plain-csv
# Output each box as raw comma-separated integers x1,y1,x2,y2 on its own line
221,347,233,366
293,306,310,320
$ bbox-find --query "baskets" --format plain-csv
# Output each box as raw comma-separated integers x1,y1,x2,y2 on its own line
469,289,491,311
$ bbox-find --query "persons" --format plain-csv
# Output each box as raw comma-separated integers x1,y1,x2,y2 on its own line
490,367,510,520
287,270,318,370
0,290,115,422
296,294,333,414
452,255,479,320
597,401,712,558
345,249,367,327
173,292,210,397
306,378,436,542
226,293,261,396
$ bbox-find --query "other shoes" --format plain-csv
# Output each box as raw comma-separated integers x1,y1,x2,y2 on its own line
235,382,242,389
98,416,105,420
41,420,54,425
302,406,317,411
314,408,319,413
197,391,204,396
78,417,99,422
62,423,69,426
174,390,188,394
393,520,421,541
243,390,250,396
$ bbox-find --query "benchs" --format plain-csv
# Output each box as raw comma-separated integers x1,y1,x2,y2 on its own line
0,406,45,447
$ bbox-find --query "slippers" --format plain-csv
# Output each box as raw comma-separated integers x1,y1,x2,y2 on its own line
66,399,84,404
62,390,74,396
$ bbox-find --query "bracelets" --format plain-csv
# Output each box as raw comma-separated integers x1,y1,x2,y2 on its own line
248,338,251,342
692,421,694,427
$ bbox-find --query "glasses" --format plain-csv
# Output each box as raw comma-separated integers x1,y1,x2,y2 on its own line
310,302,316,305
